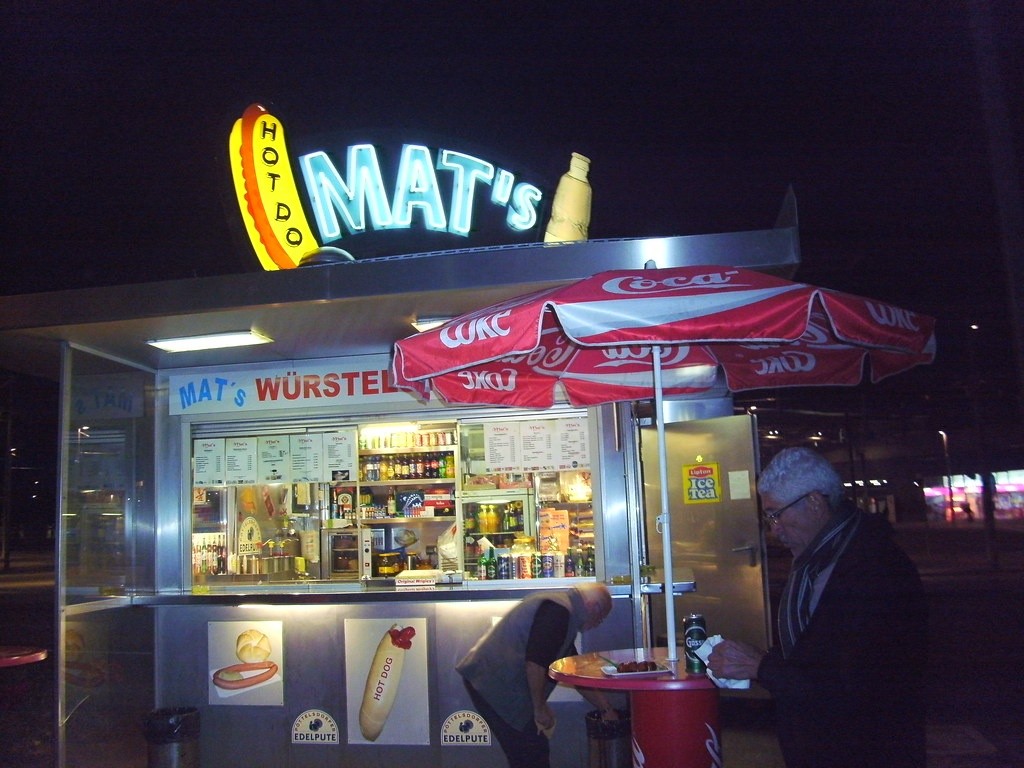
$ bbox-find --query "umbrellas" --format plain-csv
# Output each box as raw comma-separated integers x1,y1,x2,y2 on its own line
391,260,938,661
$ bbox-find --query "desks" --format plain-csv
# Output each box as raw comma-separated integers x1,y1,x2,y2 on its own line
0,550,55,591
0,645,47,667
548,646,723,768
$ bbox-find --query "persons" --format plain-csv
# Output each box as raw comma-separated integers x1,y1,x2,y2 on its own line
707,446,924,768
454,584,618,768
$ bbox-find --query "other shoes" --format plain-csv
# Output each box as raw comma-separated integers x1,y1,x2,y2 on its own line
49,568,55,572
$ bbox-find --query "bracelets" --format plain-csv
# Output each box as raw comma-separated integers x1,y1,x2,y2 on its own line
602,705,612,713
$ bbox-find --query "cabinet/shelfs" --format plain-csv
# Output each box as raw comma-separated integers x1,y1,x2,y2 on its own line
322,423,538,582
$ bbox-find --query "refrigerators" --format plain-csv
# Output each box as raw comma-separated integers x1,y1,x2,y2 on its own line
455,488,537,580
191,487,238,577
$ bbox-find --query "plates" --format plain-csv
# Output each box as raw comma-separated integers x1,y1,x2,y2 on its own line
601,664,670,677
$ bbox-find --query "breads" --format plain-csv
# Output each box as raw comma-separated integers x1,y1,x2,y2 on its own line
360,622,407,742
236,629,270,663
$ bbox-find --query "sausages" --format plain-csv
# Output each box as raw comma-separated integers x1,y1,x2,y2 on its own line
213,661,278,690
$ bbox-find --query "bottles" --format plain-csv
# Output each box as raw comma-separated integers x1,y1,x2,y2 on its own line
331,499,343,518
193,534,226,575
466,504,523,533
388,489,396,514
359,451,454,482
563,546,595,577
478,545,498,580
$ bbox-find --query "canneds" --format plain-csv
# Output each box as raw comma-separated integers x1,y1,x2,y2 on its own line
683,611,708,674
497,551,565,579
359,432,454,451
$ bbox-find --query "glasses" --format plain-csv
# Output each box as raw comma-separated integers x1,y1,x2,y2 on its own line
596,601,605,624
763,489,830,527
